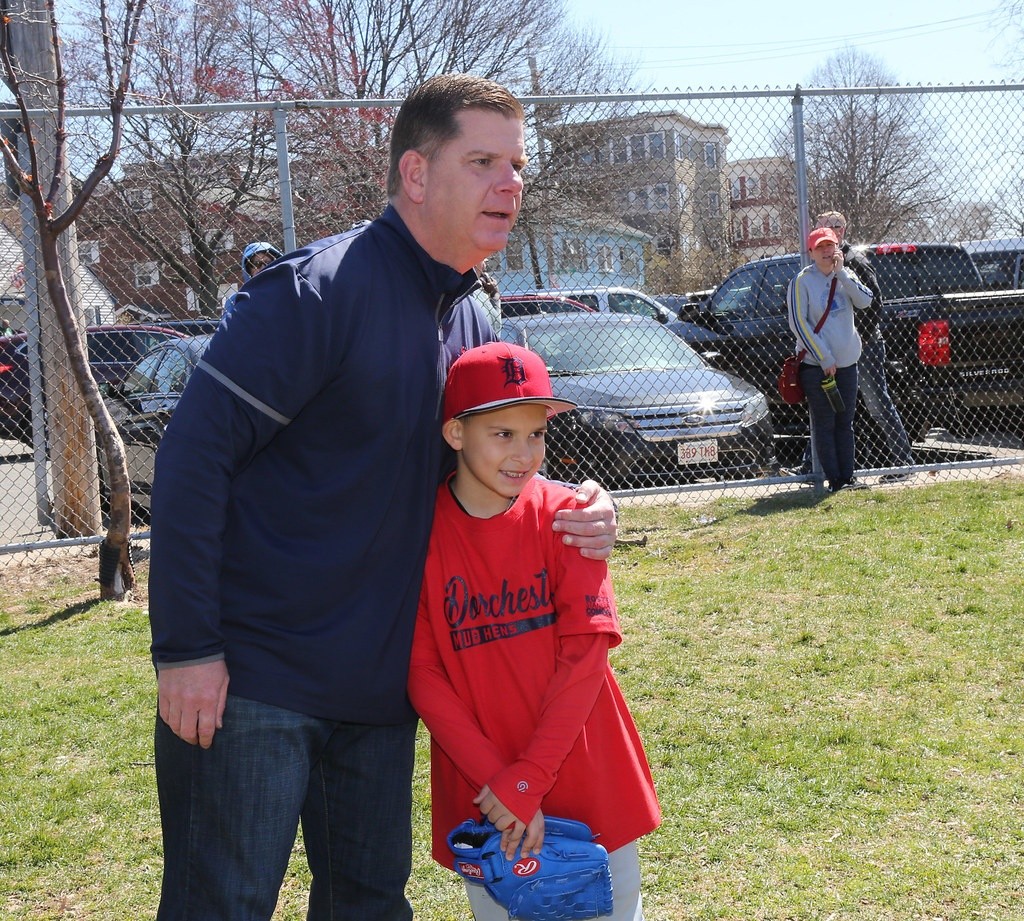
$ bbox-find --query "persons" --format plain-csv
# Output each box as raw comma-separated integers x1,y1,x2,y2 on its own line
2,319,13,336
908,296,967,444
115,303,134,325
151,73,667,921
787,211,915,495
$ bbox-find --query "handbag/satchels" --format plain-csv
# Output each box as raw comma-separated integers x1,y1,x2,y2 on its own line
778,356,804,405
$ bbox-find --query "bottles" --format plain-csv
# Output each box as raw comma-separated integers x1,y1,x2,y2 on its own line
820,376,845,414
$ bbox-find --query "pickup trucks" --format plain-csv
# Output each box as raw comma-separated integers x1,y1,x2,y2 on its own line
662,244,1024,446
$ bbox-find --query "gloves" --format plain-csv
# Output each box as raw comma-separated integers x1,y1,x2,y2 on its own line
480,272,499,297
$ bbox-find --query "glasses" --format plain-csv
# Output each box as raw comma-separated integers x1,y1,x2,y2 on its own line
249,261,271,269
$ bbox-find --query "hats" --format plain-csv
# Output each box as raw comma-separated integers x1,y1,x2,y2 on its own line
807,227,839,251
442,344,578,424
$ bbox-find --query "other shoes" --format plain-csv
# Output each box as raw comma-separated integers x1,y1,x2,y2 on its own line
827,476,867,493
879,463,917,482
779,465,815,485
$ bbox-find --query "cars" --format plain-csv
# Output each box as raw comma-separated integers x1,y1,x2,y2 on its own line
0,320,221,526
956,238,1024,291
499,287,736,366
500,312,779,492
